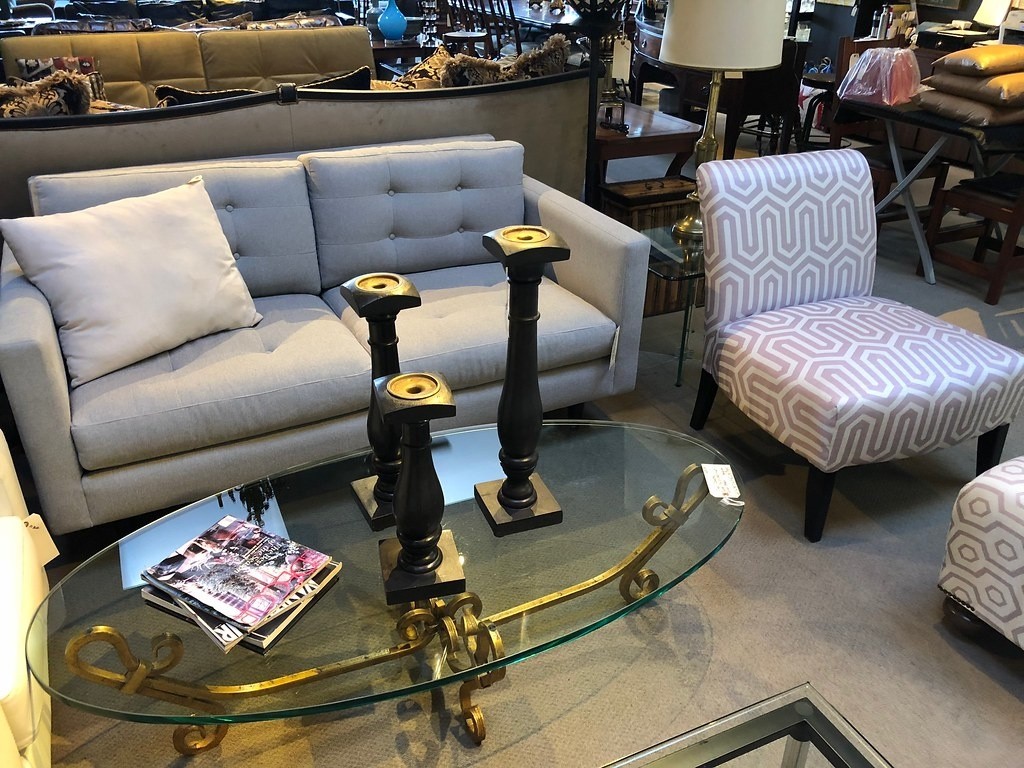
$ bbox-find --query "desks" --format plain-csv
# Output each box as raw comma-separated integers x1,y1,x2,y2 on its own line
832,101,1024,285
447,0,580,33
588,99,703,209
631,13,812,159
599,681,895,768
444,31,490,57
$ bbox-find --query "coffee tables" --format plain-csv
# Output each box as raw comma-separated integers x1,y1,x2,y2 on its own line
26,420,748,756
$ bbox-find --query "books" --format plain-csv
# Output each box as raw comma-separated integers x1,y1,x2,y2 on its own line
138,514,343,657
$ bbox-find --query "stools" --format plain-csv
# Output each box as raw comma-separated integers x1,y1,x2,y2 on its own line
790,73,852,153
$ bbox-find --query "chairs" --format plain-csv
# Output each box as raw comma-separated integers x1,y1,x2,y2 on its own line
688,149,1024,544
917,171,1024,306
828,34,949,256
441,0,542,60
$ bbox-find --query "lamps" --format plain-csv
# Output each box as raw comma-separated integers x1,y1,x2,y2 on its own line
659,0,787,242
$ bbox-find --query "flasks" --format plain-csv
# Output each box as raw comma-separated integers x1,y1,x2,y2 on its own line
871,11,882,37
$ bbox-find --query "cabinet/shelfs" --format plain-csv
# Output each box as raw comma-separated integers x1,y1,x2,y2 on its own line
824,29,1024,174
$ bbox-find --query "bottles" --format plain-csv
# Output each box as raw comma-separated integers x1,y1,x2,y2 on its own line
877,2,891,38
378,0,407,40
367,0,383,41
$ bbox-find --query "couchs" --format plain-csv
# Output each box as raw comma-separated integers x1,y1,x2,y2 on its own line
0,24,377,108
0,132,650,567
30,16,344,36
937,455,1024,661
0,0,56,21
1,67,600,219
55,0,356,27
0,427,52,768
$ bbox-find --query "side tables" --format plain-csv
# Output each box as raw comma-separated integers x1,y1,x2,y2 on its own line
640,226,706,389
0,17,52,36
369,33,443,81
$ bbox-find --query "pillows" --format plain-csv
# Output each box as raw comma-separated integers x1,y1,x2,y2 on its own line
0,68,93,118
370,79,442,91
196,11,253,30
920,72,1024,108
440,32,572,88
153,84,262,107
929,43,1024,78
5,71,107,101
0,174,263,388
15,56,95,82
397,44,452,81
298,65,372,91
